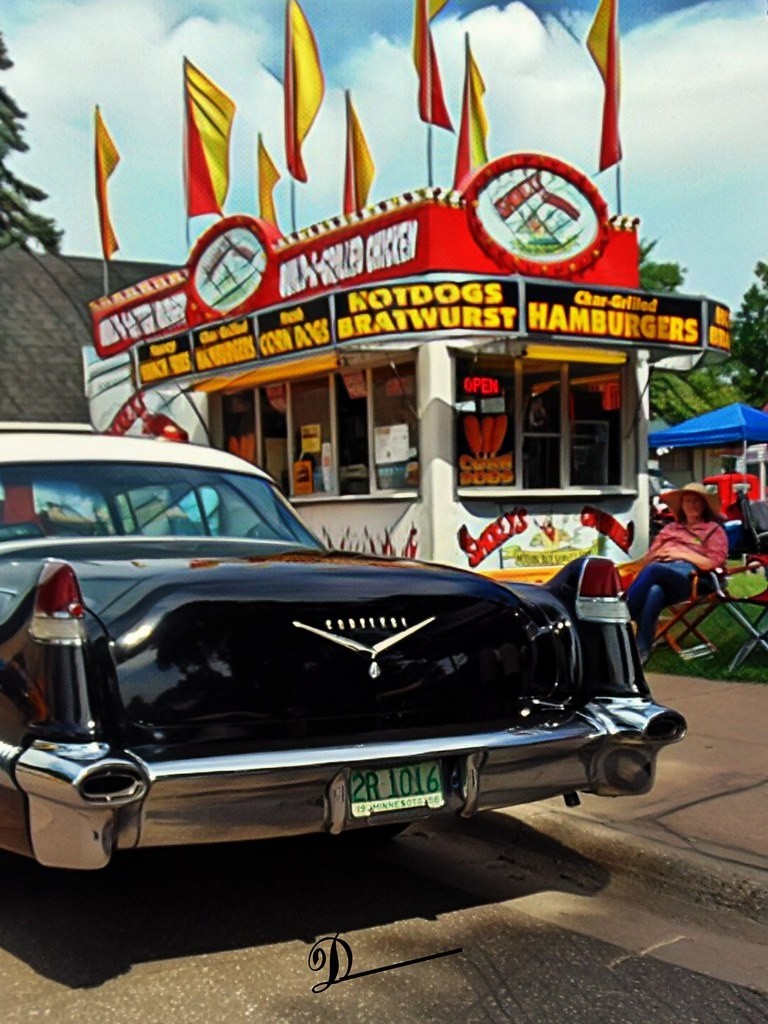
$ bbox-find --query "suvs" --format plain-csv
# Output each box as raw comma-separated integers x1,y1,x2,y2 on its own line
648,467,683,544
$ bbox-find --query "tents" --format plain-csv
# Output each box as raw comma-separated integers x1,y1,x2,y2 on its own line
647,402,767,503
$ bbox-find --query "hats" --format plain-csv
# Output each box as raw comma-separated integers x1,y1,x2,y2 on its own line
662,482,721,519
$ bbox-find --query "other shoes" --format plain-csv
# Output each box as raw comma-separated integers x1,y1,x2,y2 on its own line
639,648,650,666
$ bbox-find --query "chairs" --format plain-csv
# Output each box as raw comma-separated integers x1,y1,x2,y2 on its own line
736,491,768,550
705,553,768,675
620,561,727,667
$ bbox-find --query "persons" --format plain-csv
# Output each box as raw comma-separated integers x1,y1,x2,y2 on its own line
646,454,744,502
616,481,729,667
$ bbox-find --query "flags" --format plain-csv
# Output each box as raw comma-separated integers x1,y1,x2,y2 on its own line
182,57,237,218
342,89,376,214
410,0,456,135
257,133,281,230
586,0,624,173
283,0,327,184
453,32,488,193
93,103,120,261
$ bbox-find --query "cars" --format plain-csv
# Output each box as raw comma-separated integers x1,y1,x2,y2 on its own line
0,419,685,869
